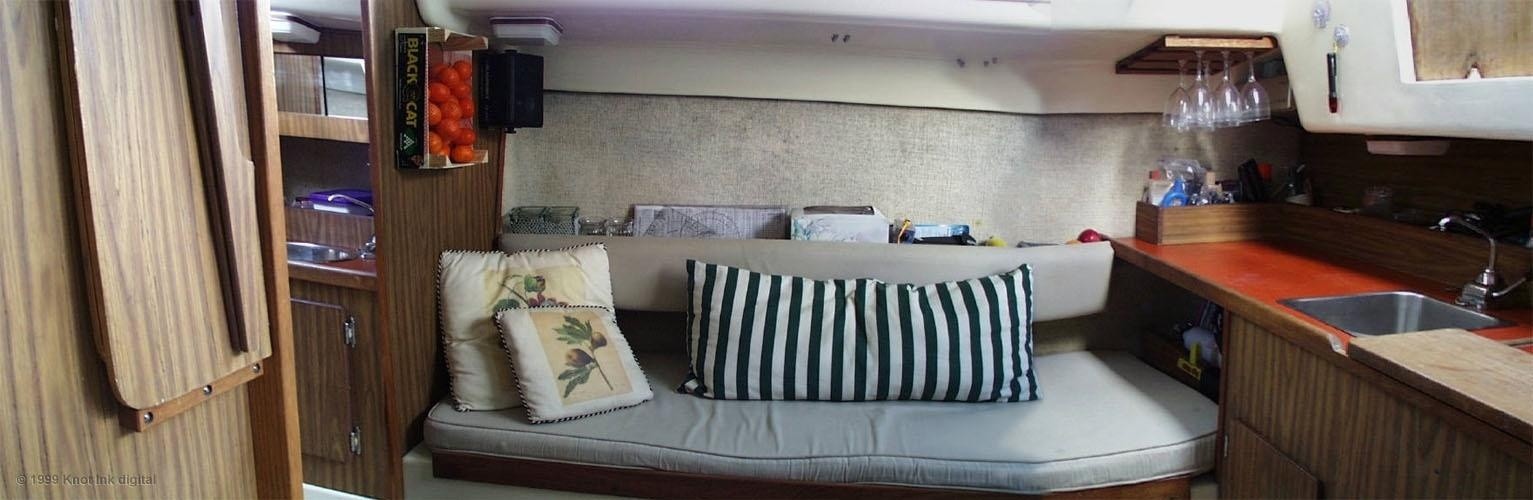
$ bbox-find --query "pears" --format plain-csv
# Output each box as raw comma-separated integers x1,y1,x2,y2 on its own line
987,235,1008,247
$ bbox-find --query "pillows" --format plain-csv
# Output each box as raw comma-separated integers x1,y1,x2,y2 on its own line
442,245,616,413
681,258,1038,401
496,305,658,421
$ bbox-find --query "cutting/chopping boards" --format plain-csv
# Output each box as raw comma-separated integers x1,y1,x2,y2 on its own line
1347,328,1533,442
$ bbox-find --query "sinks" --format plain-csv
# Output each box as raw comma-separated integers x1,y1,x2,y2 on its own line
286,240,356,264
1282,289,1497,342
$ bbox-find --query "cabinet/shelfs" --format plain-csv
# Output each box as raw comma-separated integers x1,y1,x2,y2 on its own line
268,53,409,500
1216,312,1531,499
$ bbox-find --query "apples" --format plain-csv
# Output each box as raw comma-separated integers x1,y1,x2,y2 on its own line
1068,229,1101,244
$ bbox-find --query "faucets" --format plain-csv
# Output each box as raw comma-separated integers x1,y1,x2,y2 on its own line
1437,208,1527,308
328,193,372,220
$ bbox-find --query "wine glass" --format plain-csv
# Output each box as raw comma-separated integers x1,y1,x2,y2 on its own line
1161,50,1271,131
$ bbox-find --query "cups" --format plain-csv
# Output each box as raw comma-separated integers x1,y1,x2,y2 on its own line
577,216,634,235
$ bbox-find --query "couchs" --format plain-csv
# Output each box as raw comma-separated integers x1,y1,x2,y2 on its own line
421,231,1226,500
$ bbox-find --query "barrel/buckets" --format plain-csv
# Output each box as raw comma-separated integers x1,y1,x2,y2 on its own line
1143,168,1188,206
